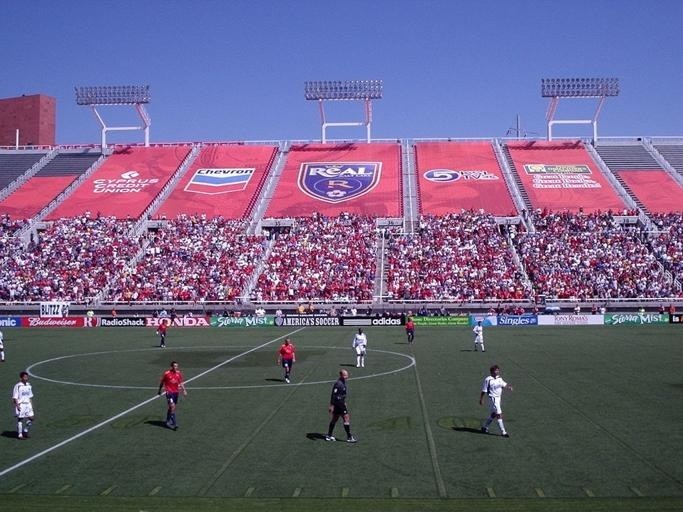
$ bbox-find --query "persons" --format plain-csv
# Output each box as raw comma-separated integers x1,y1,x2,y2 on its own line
325,369,359,443
352,328,368,368
158,361,188,430
0,331,5,362
403,317,415,344
11,372,35,440
480,365,514,437
1,205,682,319
275,338,296,384
668,304,675,323
472,321,486,352
155,319,168,348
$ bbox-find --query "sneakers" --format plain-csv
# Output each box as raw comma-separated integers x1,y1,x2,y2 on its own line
407,341,413,345
475,348,478,351
501,432,510,438
482,425,489,432
481,350,485,352
161,344,166,348
325,435,336,442
346,436,357,443
284,377,292,384
22,432,30,438
166,423,178,429
17,436,26,441
356,364,365,368
